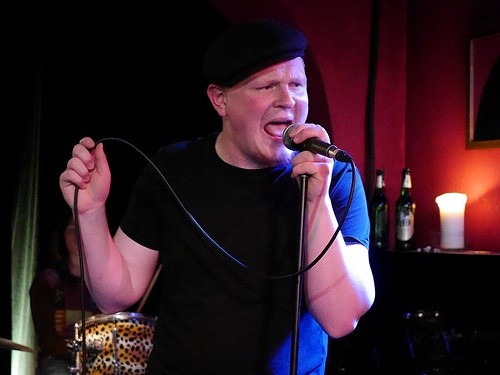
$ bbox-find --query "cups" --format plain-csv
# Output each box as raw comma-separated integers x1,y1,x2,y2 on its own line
435,191,468,250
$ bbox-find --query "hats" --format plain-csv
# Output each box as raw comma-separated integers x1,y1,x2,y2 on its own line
201,19,307,87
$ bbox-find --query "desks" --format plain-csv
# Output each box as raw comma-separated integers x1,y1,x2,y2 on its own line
362,246,500,353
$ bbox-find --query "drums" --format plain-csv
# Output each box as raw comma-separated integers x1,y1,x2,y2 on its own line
72,311,157,375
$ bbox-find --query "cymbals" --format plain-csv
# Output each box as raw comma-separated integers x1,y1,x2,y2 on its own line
0,337,39,353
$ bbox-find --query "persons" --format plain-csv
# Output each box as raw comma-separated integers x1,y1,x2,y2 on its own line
59,23,375,375
37,211,97,374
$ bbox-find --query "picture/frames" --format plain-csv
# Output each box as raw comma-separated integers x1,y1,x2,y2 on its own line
464,27,500,150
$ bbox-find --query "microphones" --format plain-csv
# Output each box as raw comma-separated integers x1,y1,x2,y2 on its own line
282,123,351,164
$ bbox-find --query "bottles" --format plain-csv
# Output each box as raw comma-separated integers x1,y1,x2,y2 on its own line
394,167,415,251
368,168,391,251
